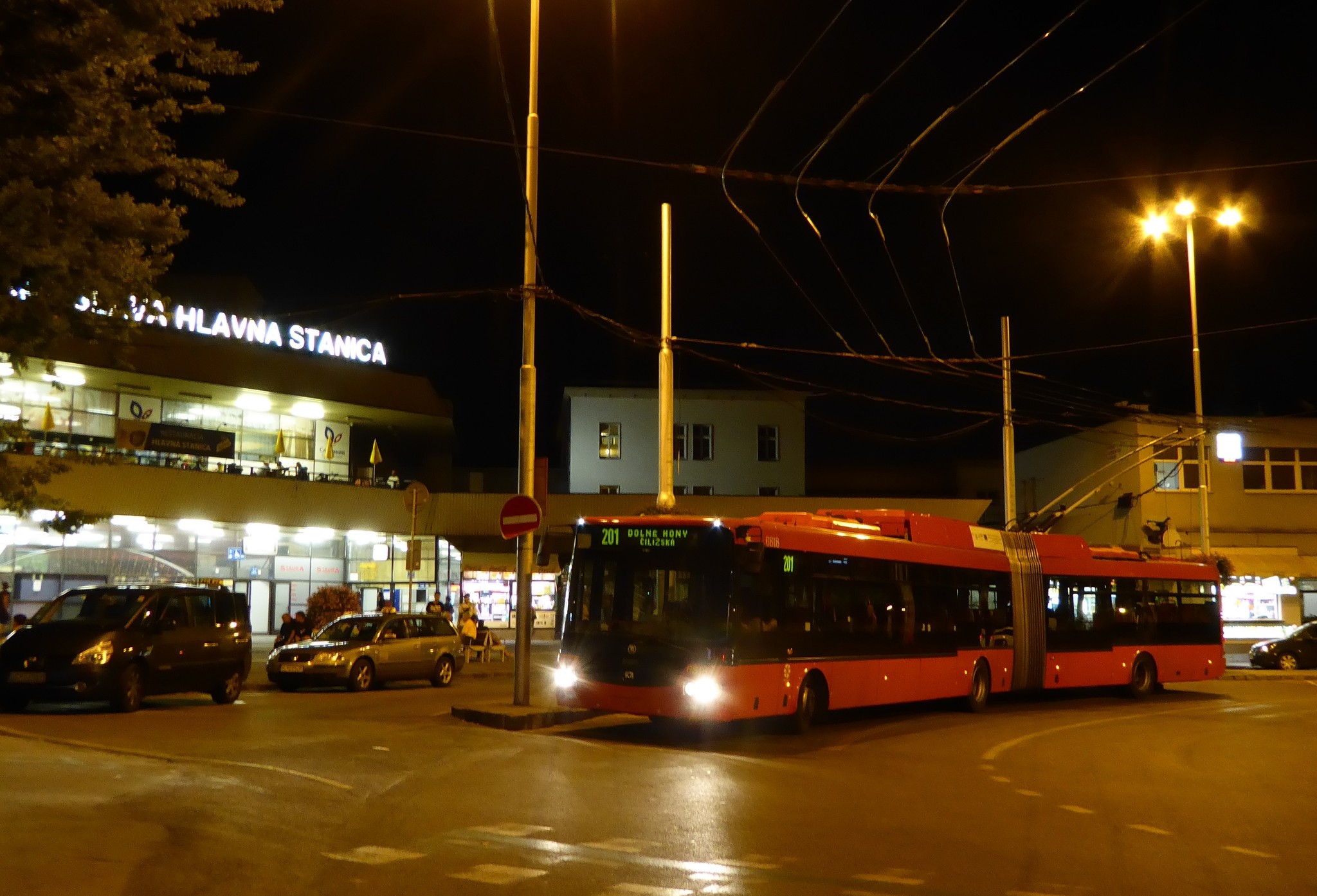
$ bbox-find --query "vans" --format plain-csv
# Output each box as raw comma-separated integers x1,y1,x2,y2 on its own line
0,581,254,714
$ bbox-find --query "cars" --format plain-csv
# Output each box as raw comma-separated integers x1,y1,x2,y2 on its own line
1249,621,1317,672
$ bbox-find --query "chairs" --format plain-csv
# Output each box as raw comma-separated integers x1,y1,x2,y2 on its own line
16,441,372,487
1115,602,1213,623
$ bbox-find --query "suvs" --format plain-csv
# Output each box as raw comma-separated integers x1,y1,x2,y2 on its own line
266,607,467,691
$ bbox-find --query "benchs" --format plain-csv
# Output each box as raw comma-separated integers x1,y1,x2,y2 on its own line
463,630,505,664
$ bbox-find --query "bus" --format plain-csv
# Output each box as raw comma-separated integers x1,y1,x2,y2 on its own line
551,505,1227,738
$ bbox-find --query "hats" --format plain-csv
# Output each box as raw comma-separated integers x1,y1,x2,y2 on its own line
464,594,470,597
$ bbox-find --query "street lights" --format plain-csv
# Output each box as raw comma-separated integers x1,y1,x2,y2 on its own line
1141,195,1250,557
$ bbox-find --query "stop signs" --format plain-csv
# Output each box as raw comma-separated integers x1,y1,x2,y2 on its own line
499,495,541,540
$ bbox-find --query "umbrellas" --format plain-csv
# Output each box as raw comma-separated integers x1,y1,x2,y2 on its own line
325,433,335,480
370,438,383,482
274,427,285,476
43,402,55,449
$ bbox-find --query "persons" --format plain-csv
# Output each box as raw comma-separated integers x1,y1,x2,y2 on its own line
296,462,308,476
0,582,11,625
388,469,400,485
258,459,284,475
375,591,386,612
214,461,224,473
426,592,511,661
273,611,313,650
